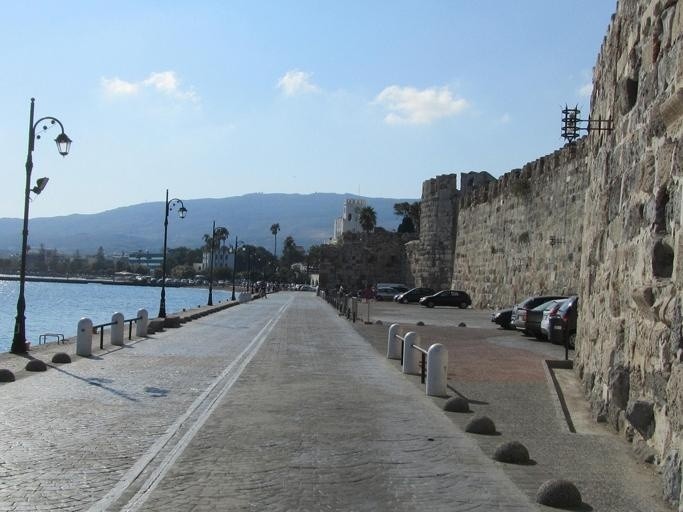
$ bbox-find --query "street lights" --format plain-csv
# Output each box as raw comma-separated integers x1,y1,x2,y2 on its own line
208,221,226,306
158,189,188,318
231,236,261,300
9,98,72,355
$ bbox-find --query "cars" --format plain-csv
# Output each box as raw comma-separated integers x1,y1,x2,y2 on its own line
492,295,577,350
151,278,231,286
301,286,316,291
419,290,472,309
371,283,436,304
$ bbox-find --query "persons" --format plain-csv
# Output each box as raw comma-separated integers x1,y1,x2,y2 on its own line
338,283,344,299
241,278,303,300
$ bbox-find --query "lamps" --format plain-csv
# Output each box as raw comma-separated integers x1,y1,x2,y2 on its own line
560,102,614,144
